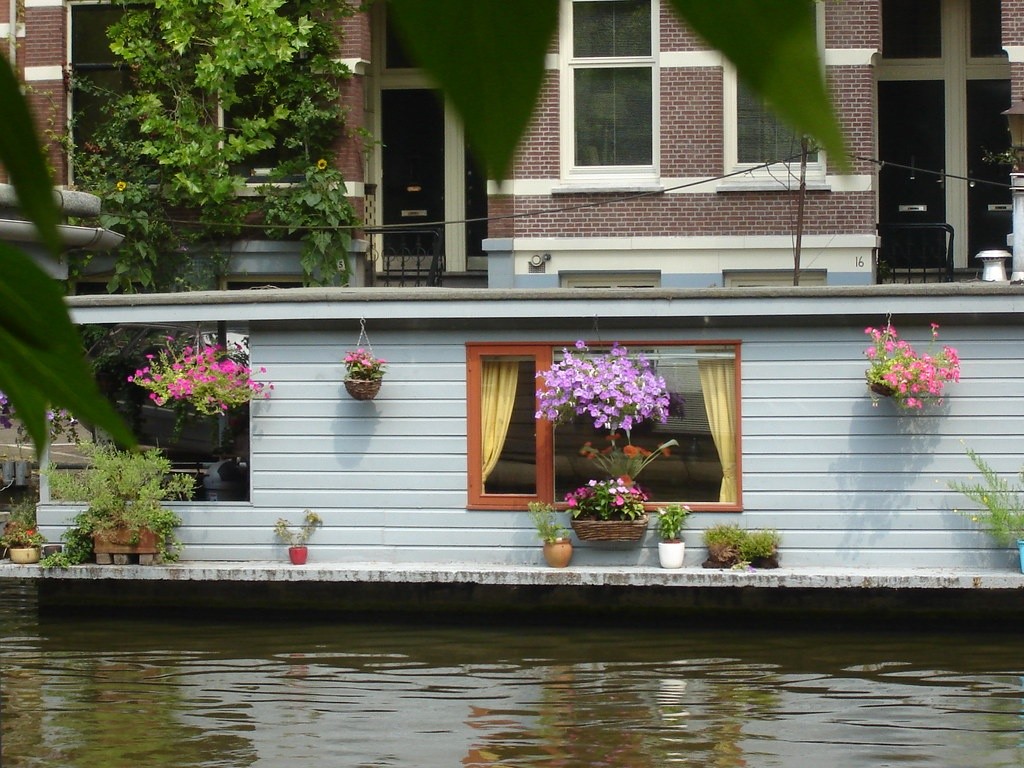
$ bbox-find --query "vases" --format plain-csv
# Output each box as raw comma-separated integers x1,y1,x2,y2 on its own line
9,547,40,564
866,373,897,398
658,541,686,569
1017,540,1024,574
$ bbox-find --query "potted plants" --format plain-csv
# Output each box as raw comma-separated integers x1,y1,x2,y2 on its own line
36,440,198,571
702,521,780,568
273,508,323,565
525,501,573,569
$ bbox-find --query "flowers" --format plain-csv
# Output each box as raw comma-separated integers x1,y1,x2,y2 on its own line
579,432,680,487
564,478,649,520
936,440,1024,544
343,348,391,382
648,506,694,542
0,522,49,548
861,322,961,418
127,337,275,433
533,340,671,430
